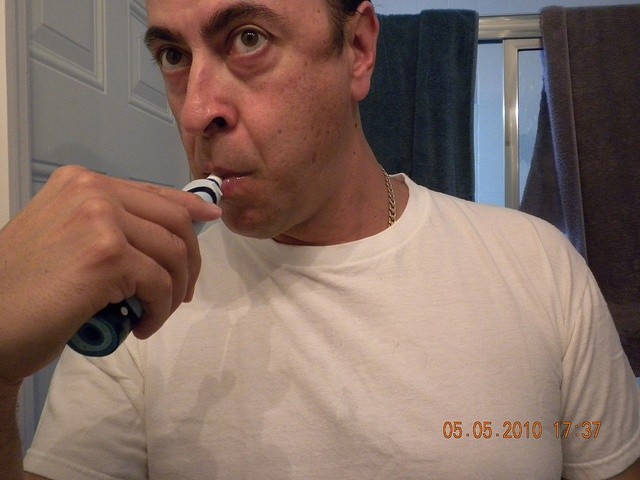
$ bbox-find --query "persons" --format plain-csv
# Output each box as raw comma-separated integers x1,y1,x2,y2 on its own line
0,0,640,480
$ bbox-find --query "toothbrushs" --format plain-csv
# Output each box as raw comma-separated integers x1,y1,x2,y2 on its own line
66,172,222,357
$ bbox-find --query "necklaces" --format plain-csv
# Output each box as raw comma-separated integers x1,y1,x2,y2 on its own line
378,163,396,231
